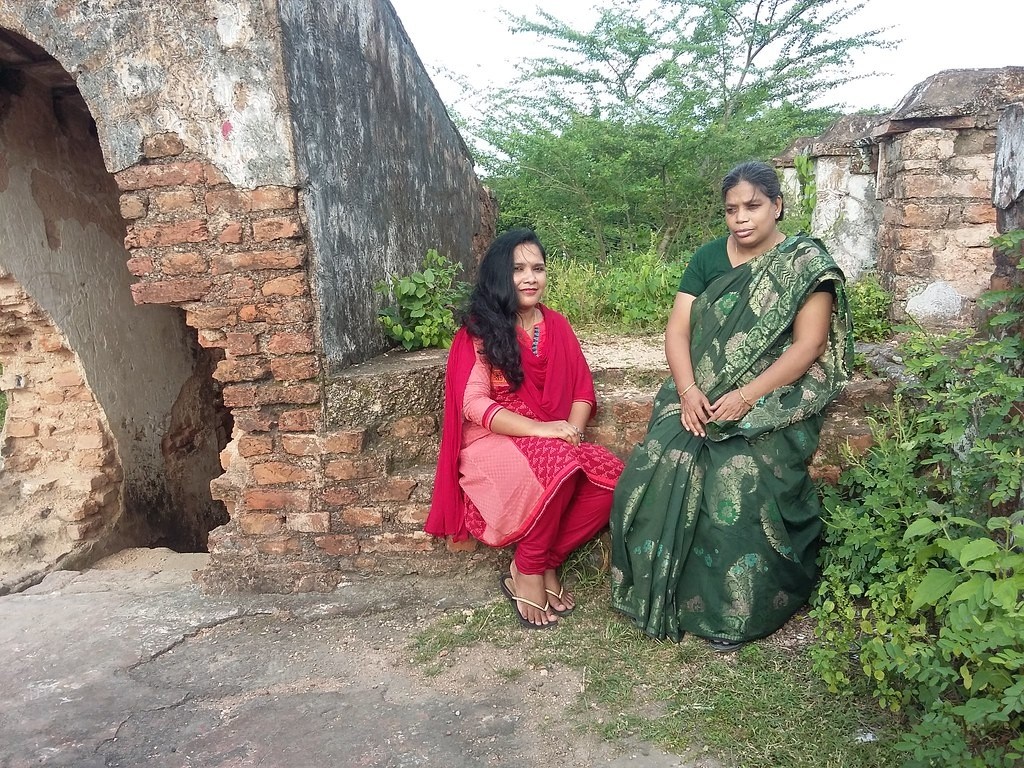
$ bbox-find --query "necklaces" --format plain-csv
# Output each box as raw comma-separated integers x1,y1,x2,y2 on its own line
525,307,537,331
735,240,778,266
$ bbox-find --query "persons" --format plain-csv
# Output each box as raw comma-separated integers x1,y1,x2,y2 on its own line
424,228,627,629
611,162,855,652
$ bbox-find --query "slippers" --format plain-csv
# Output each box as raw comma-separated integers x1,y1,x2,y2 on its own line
544,583,575,617
499,572,558,629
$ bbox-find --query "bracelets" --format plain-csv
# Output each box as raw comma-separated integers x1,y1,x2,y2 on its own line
571,425,585,442
678,382,696,397
739,389,752,407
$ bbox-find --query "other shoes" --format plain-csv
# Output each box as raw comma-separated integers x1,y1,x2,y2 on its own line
709,638,743,653
